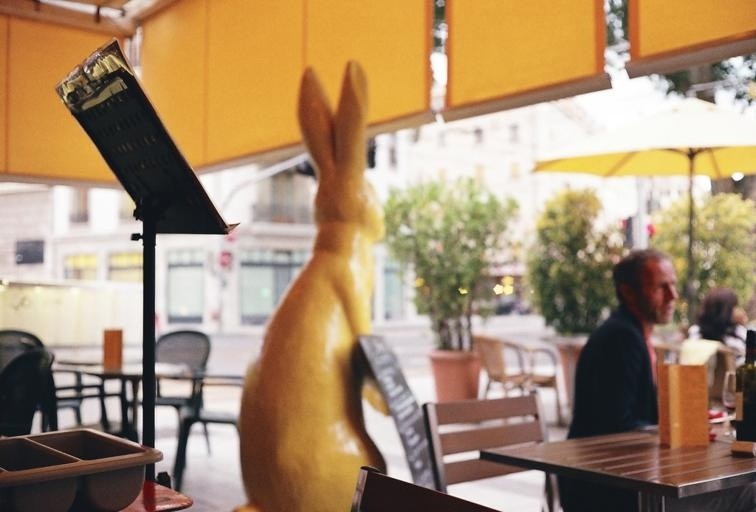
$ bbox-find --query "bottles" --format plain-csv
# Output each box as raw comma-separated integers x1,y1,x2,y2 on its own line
734,330,756,442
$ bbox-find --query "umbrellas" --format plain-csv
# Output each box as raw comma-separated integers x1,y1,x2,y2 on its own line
529,92,756,293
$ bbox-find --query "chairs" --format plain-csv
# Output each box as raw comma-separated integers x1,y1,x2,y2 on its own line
346,466,501,511
423,390,564,512
652,340,741,411
155,331,240,488
475,333,567,428
0,329,139,442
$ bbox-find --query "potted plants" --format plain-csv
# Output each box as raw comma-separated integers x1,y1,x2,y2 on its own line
529,188,634,412
377,171,521,403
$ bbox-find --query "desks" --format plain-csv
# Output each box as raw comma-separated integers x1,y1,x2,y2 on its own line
122,480,194,512
57,361,206,443
476,410,756,512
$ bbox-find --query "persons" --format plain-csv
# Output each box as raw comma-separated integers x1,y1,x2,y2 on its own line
735,307,749,342
681,289,746,366
557,247,679,512
